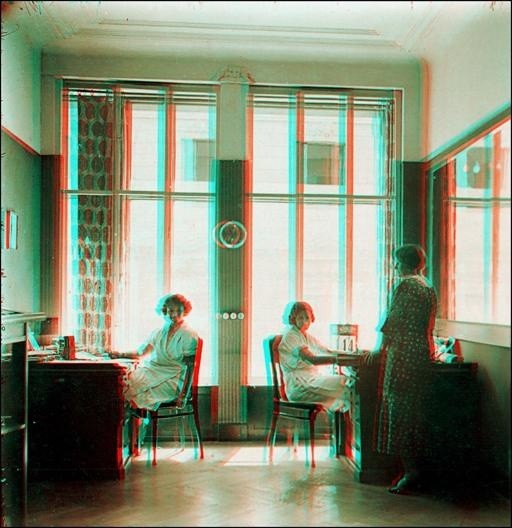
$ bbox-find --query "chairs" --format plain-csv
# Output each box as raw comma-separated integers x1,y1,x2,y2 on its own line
267,334,340,469
129,337,204,466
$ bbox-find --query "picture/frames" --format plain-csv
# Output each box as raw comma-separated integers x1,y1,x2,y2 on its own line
6,209,18,250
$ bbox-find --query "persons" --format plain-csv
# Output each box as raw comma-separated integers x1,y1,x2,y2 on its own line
277,301,354,414
362,243,437,495
109,293,198,413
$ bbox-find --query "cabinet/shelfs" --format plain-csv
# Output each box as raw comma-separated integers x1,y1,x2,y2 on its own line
28,359,145,479
0,310,47,528
339,357,477,485
